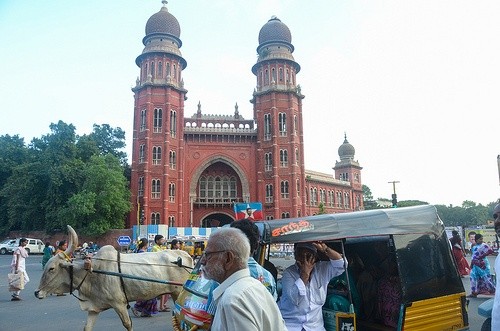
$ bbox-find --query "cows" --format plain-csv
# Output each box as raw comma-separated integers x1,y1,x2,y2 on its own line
34,224,194,331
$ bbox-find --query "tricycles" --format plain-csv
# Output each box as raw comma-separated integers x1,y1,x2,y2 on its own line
169,203,470,331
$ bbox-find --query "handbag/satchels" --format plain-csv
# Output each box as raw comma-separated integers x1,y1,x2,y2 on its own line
8,271,23,291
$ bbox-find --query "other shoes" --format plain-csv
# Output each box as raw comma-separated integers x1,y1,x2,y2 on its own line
467,295,477,298
461,277,465,278
131,307,138,317
10,293,22,301
56,294,67,296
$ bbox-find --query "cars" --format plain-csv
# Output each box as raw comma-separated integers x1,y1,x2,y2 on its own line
0,239,16,254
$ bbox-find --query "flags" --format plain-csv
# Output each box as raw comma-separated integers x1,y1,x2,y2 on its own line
234,202,263,220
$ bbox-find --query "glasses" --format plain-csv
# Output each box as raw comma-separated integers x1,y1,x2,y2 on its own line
205,250,235,258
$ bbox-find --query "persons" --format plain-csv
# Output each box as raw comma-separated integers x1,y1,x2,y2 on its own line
450,198,500,331
277,240,348,331
42,233,293,331
8,238,30,301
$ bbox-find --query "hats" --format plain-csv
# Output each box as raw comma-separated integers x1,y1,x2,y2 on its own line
295,243,317,255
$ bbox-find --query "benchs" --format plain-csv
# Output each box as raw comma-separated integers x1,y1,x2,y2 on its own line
359,287,397,331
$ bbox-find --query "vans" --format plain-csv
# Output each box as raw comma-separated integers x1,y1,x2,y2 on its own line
13,238,56,253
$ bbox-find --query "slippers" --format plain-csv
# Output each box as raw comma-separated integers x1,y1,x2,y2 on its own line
158,307,171,312
140,313,150,317
